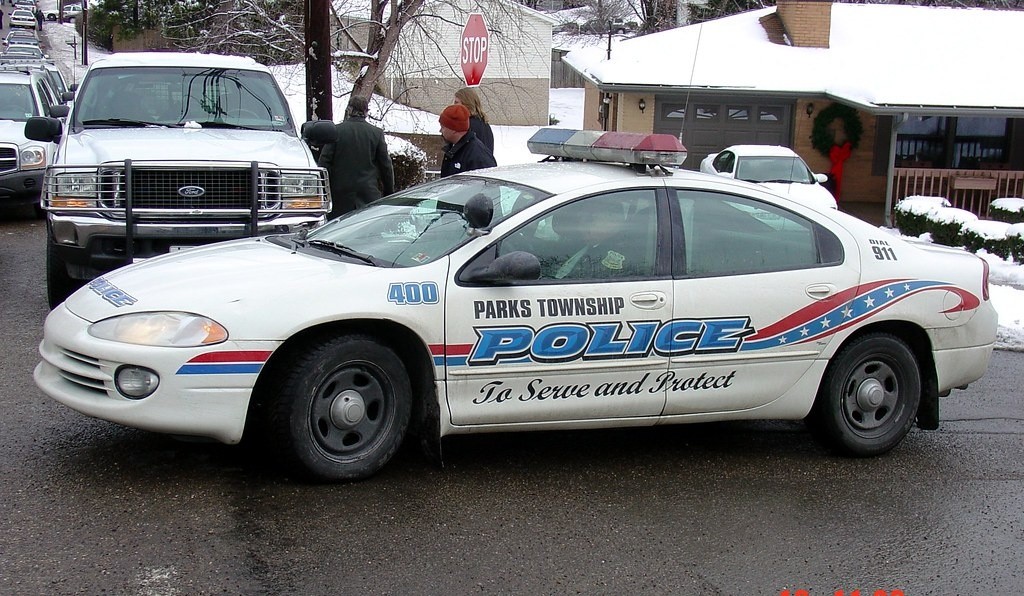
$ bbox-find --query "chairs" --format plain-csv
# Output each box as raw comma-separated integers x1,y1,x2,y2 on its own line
552,206,584,244
107,91,153,122
218,94,254,118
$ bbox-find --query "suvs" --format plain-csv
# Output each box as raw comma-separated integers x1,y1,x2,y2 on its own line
0,1,82,220
24,52,338,311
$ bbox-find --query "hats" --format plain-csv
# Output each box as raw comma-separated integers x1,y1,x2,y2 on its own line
438,105,471,132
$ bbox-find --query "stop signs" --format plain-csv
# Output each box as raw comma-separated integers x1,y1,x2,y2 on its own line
460,13,490,88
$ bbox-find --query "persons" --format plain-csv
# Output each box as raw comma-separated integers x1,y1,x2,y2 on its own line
511,192,645,279
454,87,494,155
317,95,394,221
440,104,498,179
35,10,45,31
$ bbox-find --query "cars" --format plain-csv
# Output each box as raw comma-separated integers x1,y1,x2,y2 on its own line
700,144,838,210
34,128,998,481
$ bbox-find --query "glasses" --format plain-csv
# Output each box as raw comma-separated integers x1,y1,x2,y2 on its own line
576,210,601,223
